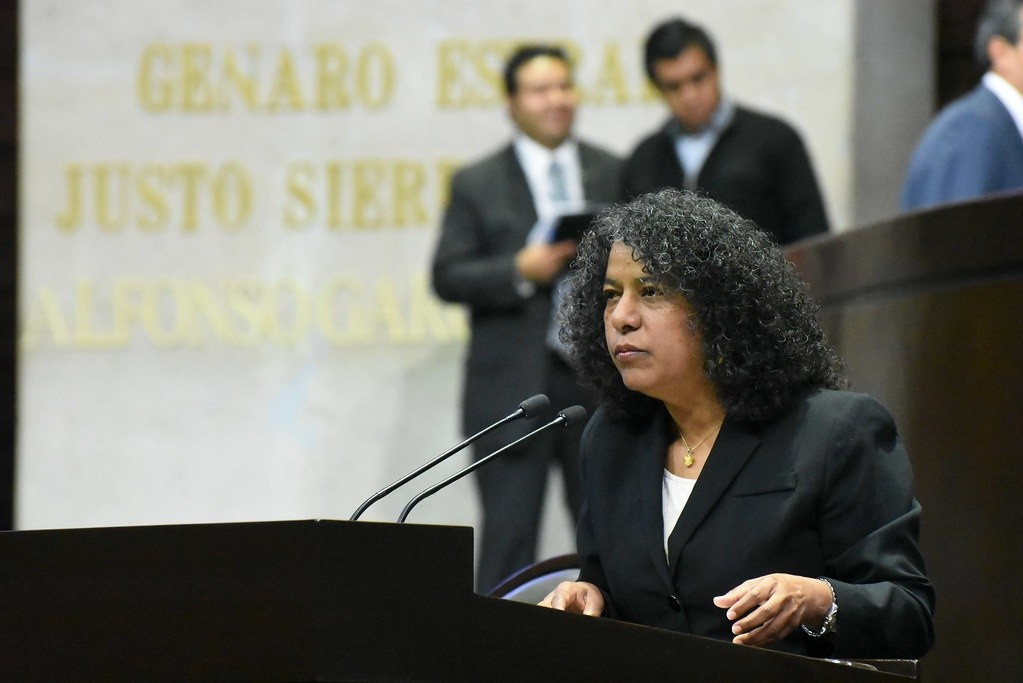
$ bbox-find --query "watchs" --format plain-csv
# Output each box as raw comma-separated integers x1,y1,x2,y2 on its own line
801,576,838,639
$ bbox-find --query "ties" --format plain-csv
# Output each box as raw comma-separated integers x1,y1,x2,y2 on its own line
549,161,570,216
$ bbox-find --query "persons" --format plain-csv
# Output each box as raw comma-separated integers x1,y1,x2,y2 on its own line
904,0,1023,210
534,190,935,661
432,41,630,597
622,18,832,250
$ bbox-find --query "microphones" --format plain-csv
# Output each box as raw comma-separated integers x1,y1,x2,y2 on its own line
349,394,552,522
395,405,587,524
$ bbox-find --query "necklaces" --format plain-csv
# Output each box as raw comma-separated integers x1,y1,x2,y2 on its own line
674,420,720,469
